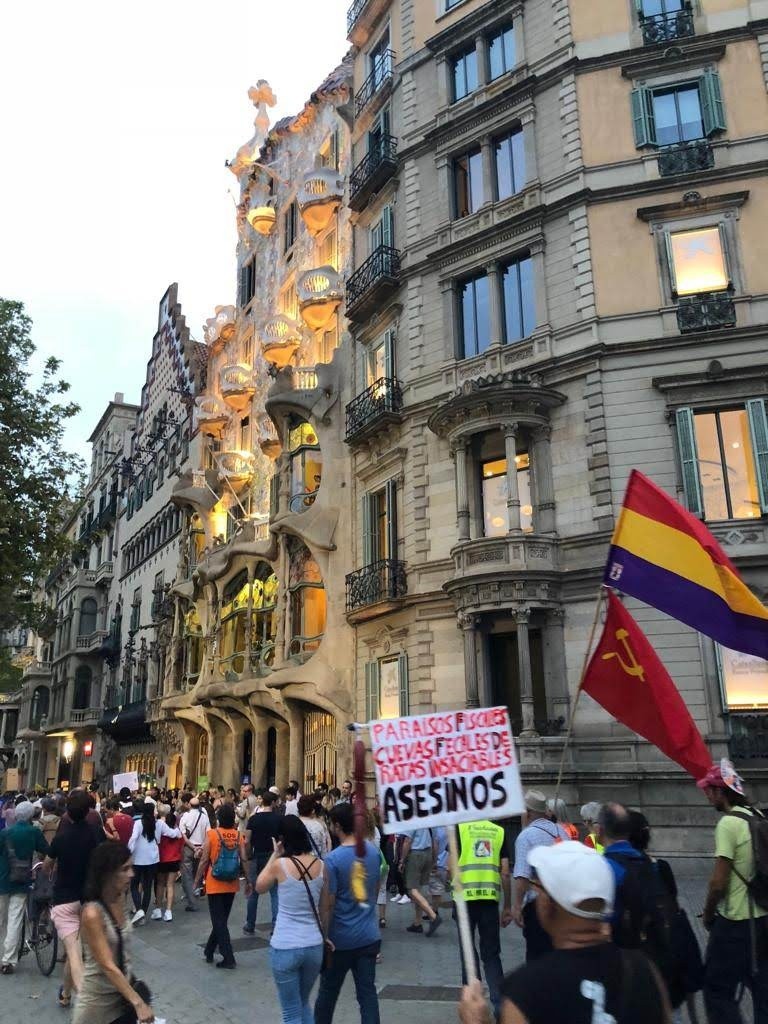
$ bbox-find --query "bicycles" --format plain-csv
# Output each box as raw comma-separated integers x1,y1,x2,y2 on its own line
16,862,58,976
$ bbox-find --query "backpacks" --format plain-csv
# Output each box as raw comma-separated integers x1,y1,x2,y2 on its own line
605,852,676,950
209,828,241,882
723,807,768,910
38,817,61,845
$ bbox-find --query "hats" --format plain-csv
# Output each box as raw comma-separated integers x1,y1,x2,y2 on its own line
523,790,547,813
526,840,615,919
696,765,725,788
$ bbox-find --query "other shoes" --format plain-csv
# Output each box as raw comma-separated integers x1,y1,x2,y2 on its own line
204,950,214,962
242,926,255,935
422,912,439,920
407,910,442,937
216,960,237,969
57,984,71,1007
185,906,195,911
1,964,13,975
378,918,388,928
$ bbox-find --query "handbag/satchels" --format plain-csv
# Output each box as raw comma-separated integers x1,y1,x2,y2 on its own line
9,859,31,884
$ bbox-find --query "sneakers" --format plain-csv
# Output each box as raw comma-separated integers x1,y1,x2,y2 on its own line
128,909,144,924
390,894,402,902
163,910,173,921
397,894,411,904
151,908,161,920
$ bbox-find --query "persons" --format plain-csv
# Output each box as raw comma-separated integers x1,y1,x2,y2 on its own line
0,758,768,1024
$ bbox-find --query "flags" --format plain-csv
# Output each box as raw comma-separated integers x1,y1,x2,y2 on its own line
580,589,714,794
600,468,768,661
